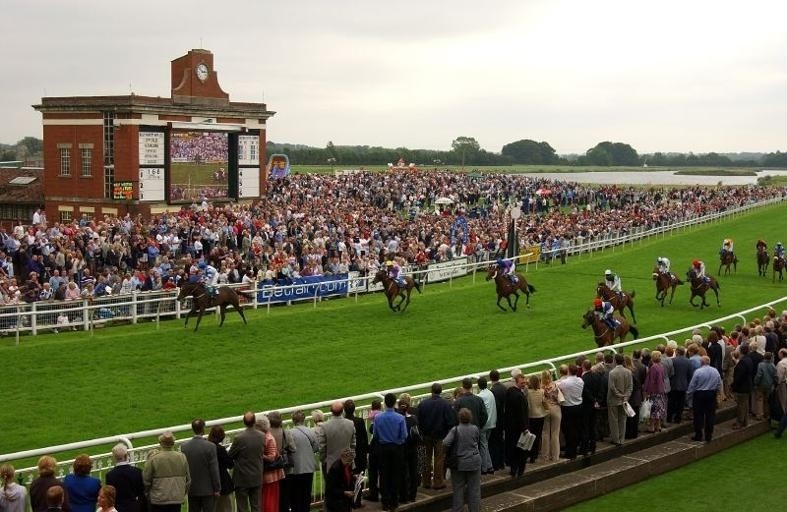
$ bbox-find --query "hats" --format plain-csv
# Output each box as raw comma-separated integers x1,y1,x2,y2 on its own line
158,430,176,446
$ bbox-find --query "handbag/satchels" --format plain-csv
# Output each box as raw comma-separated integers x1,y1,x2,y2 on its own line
265,434,285,471
554,383,566,404
410,412,423,444
443,424,459,469
280,428,295,469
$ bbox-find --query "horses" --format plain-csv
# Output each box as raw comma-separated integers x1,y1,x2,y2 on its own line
373,271,421,314
756,246,770,276
772,252,787,283
718,248,741,277
687,269,721,308
486,262,538,313
581,310,639,354
595,281,638,324
177,281,256,334
653,266,685,308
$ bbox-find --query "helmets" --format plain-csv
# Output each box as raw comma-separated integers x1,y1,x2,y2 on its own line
497,258,502,264
605,269,612,274
724,239,729,244
657,256,662,263
386,260,393,266
777,242,782,247
594,298,601,306
693,259,699,265
198,262,207,268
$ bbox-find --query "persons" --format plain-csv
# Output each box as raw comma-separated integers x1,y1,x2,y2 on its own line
502,173,558,259
106,444,147,512
65,455,102,512
385,260,402,284
0,463,32,512
363,407,393,501
774,242,785,262
476,376,496,474
558,179,787,241
311,409,327,482
524,375,547,463
267,411,296,512
540,370,561,463
604,269,622,298
583,324,725,458
369,400,382,433
451,387,464,408
181,419,222,511
0,197,262,288
692,259,707,282
454,379,488,428
445,409,482,512
373,393,408,511
489,370,507,471
387,162,499,264
344,399,368,509
576,354,585,376
95,485,118,512
30,456,69,512
290,409,319,512
555,364,570,451
46,485,67,512
497,256,516,282
261,166,385,275
142,432,191,512
724,239,734,255
657,257,671,276
208,425,236,512
593,299,616,327
318,401,355,472
505,374,529,477
756,239,768,257
254,413,286,512
505,368,527,396
398,400,423,504
558,365,584,459
228,412,266,512
417,384,454,490
399,392,415,415
326,448,356,511
724,310,787,439
